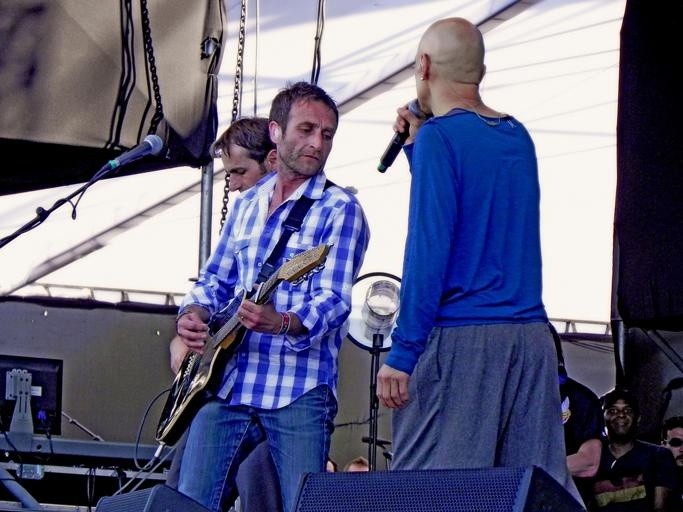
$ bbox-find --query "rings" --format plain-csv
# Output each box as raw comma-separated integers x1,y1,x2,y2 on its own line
239,315,245,323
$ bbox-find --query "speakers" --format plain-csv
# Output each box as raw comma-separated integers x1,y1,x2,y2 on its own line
95,484,211,512
291,465,586,512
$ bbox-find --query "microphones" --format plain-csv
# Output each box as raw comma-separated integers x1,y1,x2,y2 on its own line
378,98,425,173
103,134,164,171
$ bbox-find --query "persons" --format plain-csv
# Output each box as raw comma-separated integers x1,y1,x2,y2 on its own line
546,321,605,512
342,456,371,472
326,459,337,473
166,116,282,512
592,387,678,512
374,17,587,508
175,78,371,509
659,414,682,512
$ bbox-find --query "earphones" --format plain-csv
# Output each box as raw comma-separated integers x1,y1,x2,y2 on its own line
418,62,424,74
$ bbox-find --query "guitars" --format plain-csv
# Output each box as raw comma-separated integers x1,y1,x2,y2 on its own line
155,243,329,447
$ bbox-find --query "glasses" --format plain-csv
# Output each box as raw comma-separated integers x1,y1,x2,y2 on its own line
664,438,683,447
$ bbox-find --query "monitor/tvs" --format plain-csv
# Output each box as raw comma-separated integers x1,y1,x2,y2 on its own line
0,354,63,435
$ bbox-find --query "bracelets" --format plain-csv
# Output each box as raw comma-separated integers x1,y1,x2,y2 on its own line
272,312,293,337
